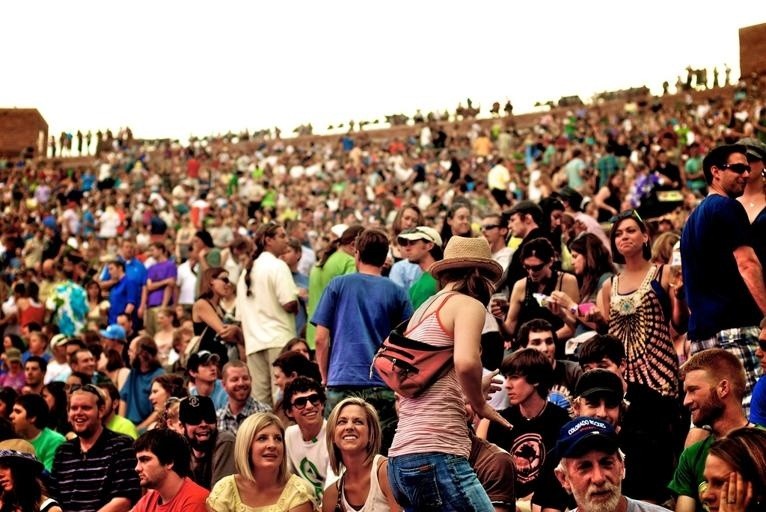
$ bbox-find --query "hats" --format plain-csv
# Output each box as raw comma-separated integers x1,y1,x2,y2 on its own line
556,416,618,456
0,439,44,476
397,226,442,248
549,187,583,212
7,348,22,363
575,369,624,400
50,334,71,348
100,326,127,339
187,350,221,369
501,201,544,221
429,235,503,286
180,395,215,419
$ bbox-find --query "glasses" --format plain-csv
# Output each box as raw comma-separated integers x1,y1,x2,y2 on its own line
70,383,101,397
218,276,229,284
609,210,644,225
480,225,500,230
523,263,545,272
185,418,216,425
291,394,321,409
721,164,753,174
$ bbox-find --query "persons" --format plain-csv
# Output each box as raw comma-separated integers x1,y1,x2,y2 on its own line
0,58,766,512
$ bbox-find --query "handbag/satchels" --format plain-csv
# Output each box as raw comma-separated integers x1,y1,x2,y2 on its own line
374,330,454,399
180,336,203,368
